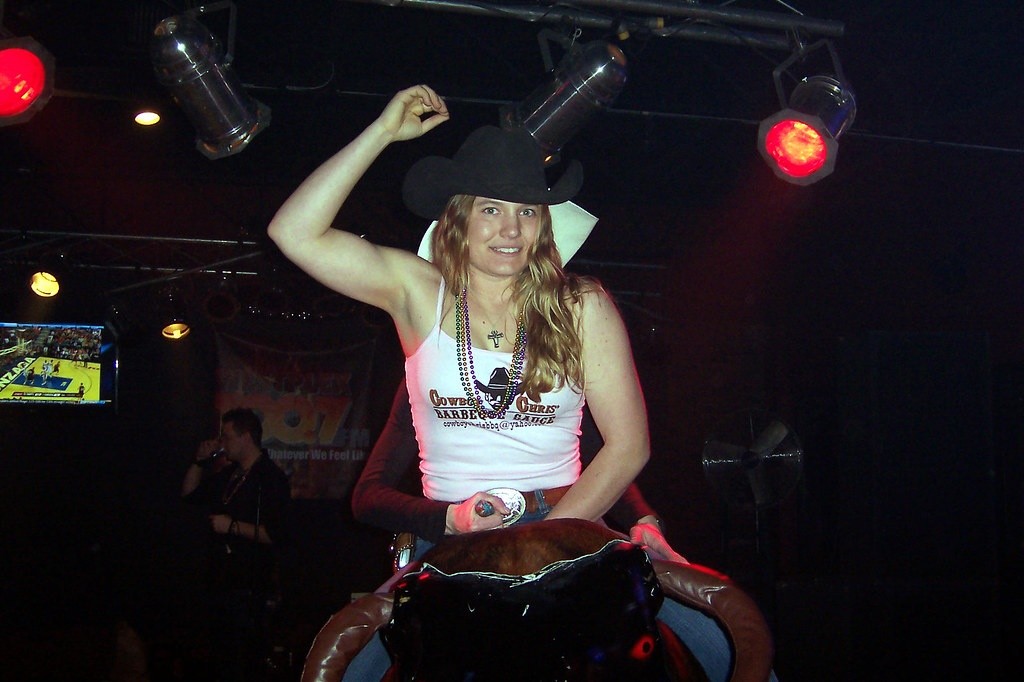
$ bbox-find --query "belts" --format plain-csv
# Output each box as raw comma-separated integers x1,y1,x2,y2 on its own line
435,484,572,521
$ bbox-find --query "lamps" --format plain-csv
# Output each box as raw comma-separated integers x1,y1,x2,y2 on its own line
150,4,273,161
1,25,58,131
498,28,630,168
756,41,857,186
152,277,195,343
28,251,74,304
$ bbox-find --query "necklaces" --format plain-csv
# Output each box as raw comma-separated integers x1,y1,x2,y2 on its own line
454,282,535,420
222,465,249,505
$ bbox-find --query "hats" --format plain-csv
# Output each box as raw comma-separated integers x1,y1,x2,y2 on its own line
401,124,584,222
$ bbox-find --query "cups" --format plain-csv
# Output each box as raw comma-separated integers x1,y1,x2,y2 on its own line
204,444,228,470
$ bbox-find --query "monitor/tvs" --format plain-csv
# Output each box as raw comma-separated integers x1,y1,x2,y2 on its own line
1,317,118,410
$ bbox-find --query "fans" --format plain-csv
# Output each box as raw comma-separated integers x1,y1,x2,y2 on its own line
697,410,803,609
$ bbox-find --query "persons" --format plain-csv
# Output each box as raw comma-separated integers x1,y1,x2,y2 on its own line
265,79,737,682
180,406,290,681
352,373,688,568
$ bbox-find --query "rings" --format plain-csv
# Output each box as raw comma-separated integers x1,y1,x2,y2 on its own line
475,500,497,518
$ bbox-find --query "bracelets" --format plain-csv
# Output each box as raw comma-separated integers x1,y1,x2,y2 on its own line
227,517,238,535
